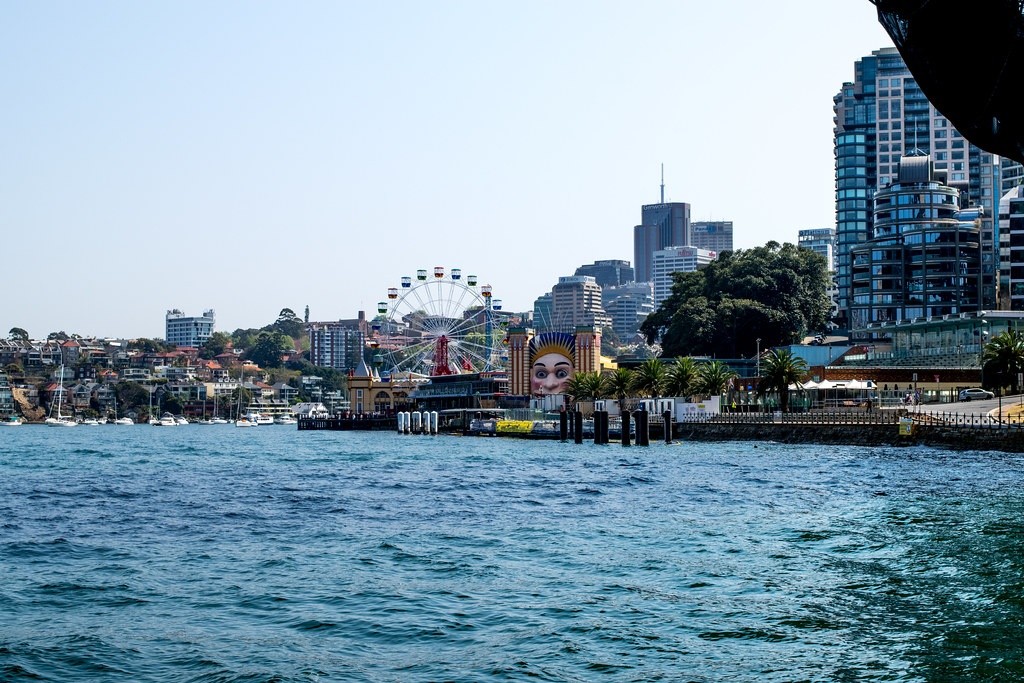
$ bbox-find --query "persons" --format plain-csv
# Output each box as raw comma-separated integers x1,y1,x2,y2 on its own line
864,397,873,413
915,391,920,405
909,394,913,406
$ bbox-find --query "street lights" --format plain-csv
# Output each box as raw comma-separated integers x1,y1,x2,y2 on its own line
756,337,761,378
980,310,988,389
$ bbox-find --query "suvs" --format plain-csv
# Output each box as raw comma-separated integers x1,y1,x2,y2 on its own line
958,388,995,402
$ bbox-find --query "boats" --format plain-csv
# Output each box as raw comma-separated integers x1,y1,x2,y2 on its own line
0,416,23,426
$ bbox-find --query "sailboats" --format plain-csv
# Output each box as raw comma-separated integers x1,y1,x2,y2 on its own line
44,363,297,427
